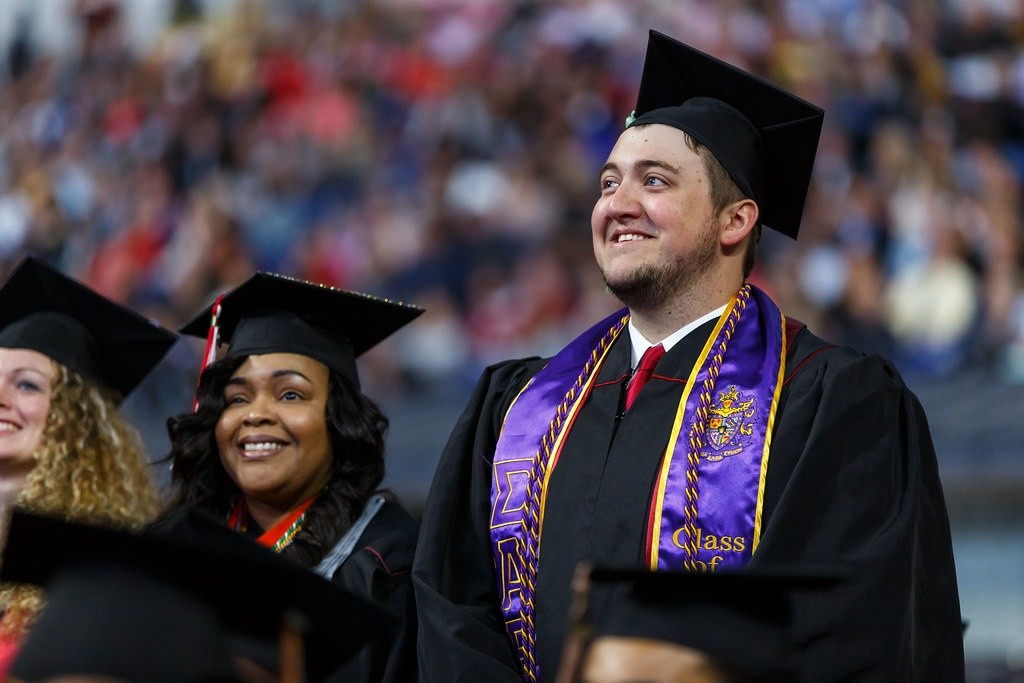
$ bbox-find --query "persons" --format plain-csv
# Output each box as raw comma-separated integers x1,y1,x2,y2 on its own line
0,254,185,683
10,270,428,683
413,27,968,682
0,0,1023,416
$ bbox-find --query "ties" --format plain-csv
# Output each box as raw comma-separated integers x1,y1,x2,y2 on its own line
625,344,667,408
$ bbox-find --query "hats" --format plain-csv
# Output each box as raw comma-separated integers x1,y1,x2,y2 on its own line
177,269,427,417
1,504,394,683
1,250,181,411
556,553,866,681
625,28,825,245
